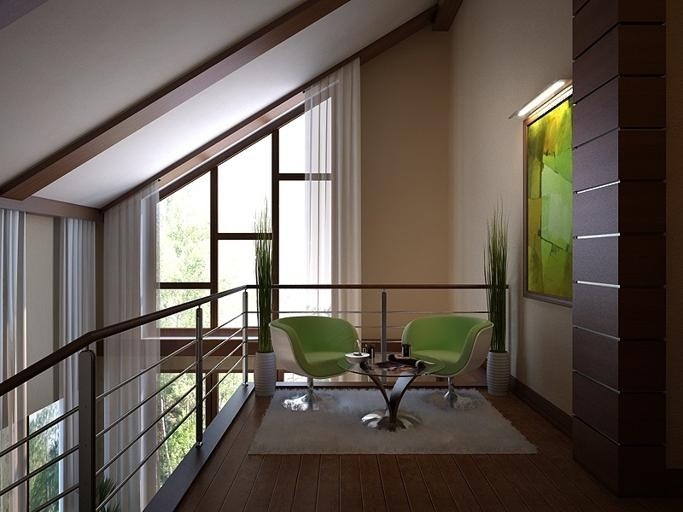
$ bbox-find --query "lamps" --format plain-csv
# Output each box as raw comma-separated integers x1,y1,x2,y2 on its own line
503,77,571,120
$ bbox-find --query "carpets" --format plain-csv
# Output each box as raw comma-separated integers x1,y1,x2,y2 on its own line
245,386,539,456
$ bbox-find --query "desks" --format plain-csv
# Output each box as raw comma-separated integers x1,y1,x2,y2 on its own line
335,350,448,436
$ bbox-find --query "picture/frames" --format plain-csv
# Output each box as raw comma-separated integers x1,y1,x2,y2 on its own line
521,84,573,311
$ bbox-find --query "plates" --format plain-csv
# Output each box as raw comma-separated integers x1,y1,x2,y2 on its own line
344,352,370,359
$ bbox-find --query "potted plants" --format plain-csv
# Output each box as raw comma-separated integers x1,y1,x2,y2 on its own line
482,204,517,399
250,207,282,400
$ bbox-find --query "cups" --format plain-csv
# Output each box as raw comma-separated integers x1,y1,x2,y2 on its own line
401,344,412,357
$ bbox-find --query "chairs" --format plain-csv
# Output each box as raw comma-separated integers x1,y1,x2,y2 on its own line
399,314,493,411
267,314,363,412
